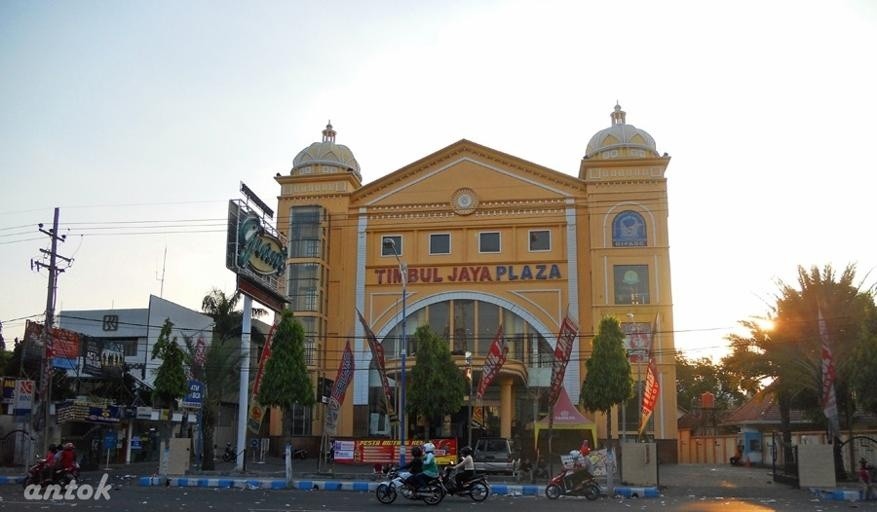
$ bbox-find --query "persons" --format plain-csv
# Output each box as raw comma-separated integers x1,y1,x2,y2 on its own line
858,465,872,500
407,441,436,494
561,449,586,491
61,442,78,478
39,443,57,467
447,446,474,497
53,444,65,468
733,439,743,464
392,445,425,495
728,452,737,465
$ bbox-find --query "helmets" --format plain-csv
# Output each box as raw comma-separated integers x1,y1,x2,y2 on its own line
570,450,581,459
412,447,423,456
581,447,591,456
460,447,473,457
64,443,75,451
423,443,436,453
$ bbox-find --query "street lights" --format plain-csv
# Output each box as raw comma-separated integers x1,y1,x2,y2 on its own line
383,238,409,469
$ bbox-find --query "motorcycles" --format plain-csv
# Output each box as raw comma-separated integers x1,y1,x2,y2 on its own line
376,461,489,506
545,462,601,500
23,455,79,494
223,445,236,463
